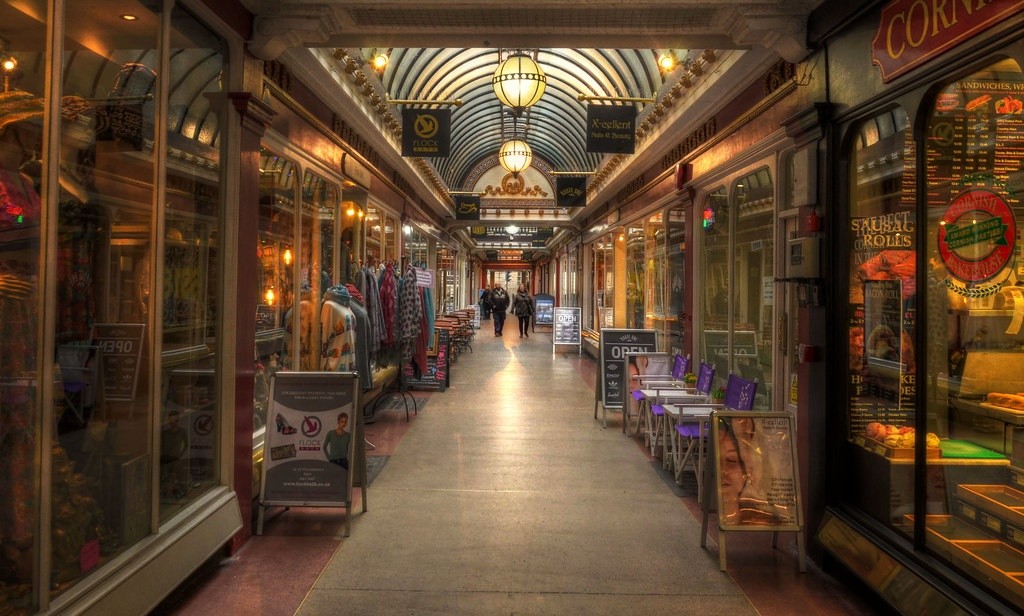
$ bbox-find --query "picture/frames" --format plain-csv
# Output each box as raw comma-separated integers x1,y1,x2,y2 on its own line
426,330,438,356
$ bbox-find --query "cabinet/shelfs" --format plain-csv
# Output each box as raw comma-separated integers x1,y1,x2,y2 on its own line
160,351,221,527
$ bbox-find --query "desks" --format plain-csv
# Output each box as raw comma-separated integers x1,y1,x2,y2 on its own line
640,390,688,456
849,439,1011,527
662,404,713,486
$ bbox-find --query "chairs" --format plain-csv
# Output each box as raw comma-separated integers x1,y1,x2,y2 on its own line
434,309,475,365
632,354,760,503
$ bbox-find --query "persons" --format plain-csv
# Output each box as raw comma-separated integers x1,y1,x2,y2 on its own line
510,283,535,338
927,464,947,504
478,287,492,320
489,283,510,337
0,89,122,590
718,416,764,525
320,284,375,390
627,280,644,329
323,412,350,469
161,411,188,462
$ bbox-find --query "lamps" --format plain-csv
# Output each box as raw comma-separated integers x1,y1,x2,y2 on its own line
359,53,388,67
492,49,547,118
658,56,688,71
504,225,521,237
499,108,532,179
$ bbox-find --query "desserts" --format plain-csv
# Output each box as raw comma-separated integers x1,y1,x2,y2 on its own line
867,422,940,448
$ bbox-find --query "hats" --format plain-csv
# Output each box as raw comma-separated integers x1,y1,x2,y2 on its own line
0,91,44,130
62,96,98,121
